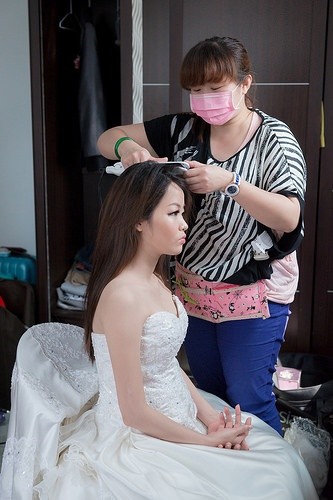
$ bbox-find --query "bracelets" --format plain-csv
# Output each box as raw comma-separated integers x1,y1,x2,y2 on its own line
115,136,132,159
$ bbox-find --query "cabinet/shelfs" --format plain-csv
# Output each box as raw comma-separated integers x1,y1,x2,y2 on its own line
28,0,333,352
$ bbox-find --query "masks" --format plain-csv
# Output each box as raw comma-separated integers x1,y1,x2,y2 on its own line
188,82,246,127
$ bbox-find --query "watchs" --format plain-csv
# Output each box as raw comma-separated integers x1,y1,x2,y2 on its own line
220,171,242,197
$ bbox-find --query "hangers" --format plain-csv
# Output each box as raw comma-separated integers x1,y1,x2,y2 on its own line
59,0,91,30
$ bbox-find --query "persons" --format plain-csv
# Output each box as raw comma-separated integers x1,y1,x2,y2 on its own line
97,37,305,435
0,162,330,500
